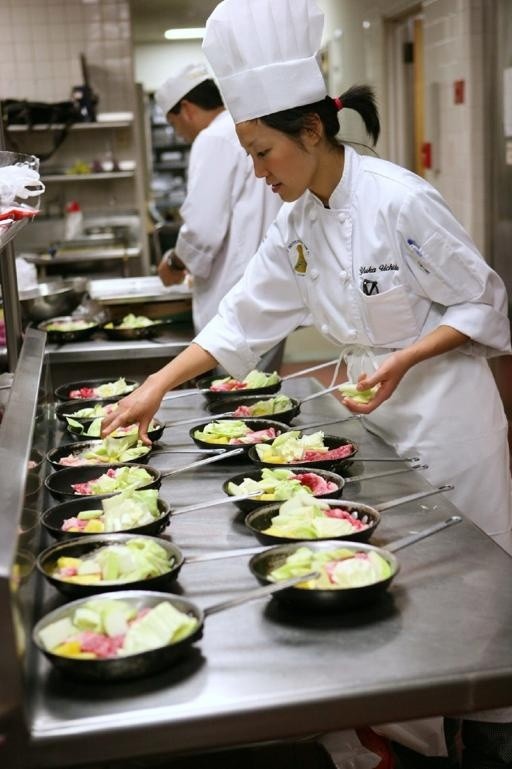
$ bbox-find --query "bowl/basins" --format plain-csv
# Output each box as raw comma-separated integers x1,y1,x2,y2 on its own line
16,276,88,320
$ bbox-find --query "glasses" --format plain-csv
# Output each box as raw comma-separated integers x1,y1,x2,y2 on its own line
361,277,378,295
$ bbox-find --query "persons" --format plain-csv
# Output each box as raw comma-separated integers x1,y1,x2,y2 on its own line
98,0,510,769
152,60,284,380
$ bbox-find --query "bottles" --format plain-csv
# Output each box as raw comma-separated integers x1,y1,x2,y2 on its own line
65,204,84,241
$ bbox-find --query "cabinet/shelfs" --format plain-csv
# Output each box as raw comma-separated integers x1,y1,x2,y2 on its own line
0,111,134,185
148,117,190,173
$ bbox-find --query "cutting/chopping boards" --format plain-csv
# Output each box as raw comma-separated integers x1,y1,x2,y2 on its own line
84,273,190,306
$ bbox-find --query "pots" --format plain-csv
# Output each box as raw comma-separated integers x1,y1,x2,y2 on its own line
32,312,176,340
25,533,304,594
224,465,429,513
250,431,423,471
45,371,258,532
190,360,368,458
249,510,465,607
247,481,454,549
25,565,326,690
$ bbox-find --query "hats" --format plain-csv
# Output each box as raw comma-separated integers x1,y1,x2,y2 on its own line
202,0,328,127
154,58,215,117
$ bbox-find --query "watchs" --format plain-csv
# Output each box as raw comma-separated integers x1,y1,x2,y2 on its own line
165,253,184,273
